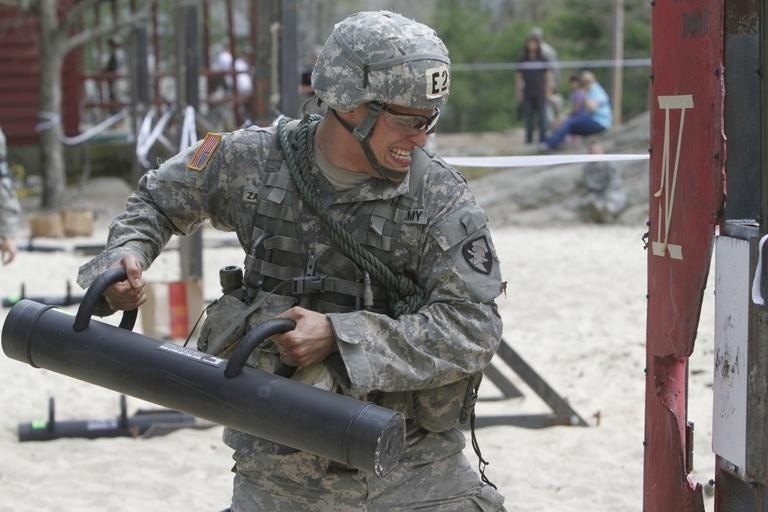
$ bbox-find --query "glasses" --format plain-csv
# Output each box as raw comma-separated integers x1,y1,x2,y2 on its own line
367,102,441,136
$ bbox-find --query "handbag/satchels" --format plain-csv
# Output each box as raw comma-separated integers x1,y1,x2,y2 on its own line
381,297,485,431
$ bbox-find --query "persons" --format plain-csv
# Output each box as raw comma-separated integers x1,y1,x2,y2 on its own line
0,130,20,267
218,38,232,97
78,12,505,512
515,28,613,154
233,47,253,122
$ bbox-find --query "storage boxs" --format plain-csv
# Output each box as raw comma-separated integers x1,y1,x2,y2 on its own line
59,210,97,237
29,210,63,239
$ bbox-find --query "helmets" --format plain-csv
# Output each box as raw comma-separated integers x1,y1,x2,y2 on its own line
308,7,452,114
